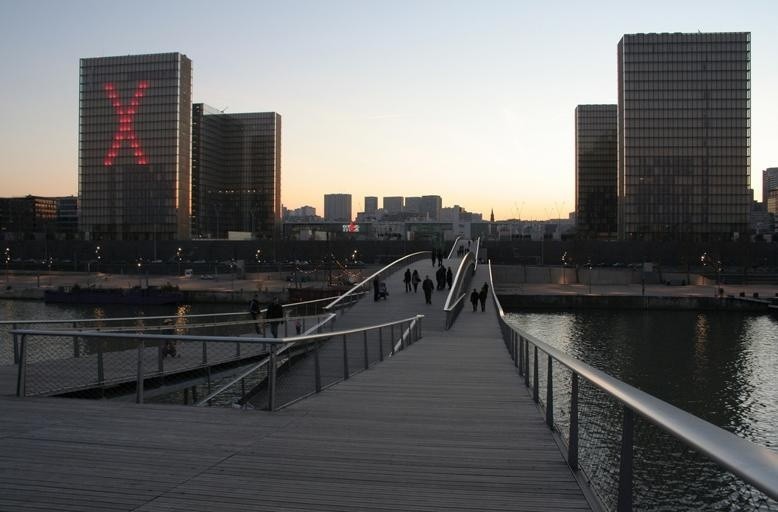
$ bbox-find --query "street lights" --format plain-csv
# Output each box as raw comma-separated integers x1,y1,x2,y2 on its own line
176,247,185,275
715,259,724,294
135,256,150,288
699,252,709,285
95,246,104,273
582,256,593,295
253,247,267,280
42,256,53,285
560,249,570,284
4,247,12,282
350,248,363,273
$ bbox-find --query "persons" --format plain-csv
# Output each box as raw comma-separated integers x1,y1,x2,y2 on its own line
470,288,479,311
373,276,379,302
404,247,453,304
248,293,262,334
266,296,284,338
479,281,489,312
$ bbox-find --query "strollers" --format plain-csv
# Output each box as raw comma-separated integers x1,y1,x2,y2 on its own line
379,283,389,300
